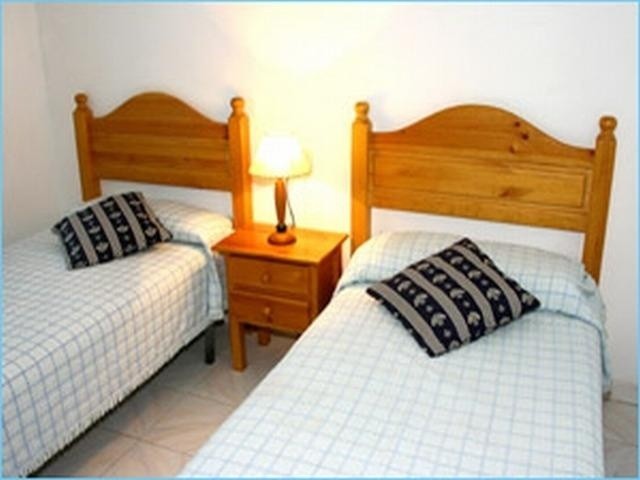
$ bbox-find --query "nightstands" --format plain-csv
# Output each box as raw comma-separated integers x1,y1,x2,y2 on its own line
211,223,349,372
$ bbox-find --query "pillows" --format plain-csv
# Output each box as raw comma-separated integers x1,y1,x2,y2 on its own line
349,231,588,316
51,191,173,272
81,190,232,245
366,237,541,357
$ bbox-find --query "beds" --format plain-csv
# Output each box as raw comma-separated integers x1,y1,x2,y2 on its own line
176,101,617,480
1,91,249,479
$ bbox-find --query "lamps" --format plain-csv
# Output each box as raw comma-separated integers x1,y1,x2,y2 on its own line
248,131,311,245
